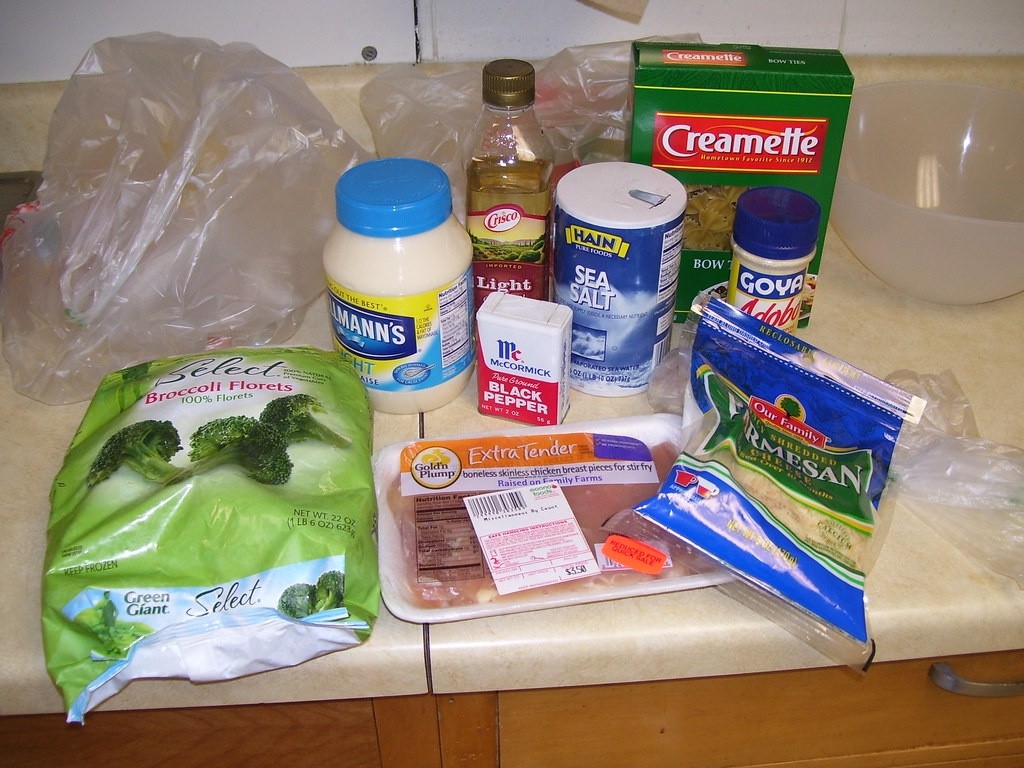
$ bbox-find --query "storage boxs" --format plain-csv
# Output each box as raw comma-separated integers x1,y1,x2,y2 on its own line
476,290,575,428
628,40,856,324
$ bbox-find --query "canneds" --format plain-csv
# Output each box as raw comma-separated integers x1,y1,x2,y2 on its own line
726,186,821,341
551,162,687,398
321,158,477,414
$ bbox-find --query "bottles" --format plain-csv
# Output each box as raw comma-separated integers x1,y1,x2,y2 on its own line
459,59,555,320
725,186,821,337
322,157,473,416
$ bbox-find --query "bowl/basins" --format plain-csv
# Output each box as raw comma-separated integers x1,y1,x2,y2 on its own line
828,80,1024,307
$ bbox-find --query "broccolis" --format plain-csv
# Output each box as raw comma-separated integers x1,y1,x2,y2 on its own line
84,393,352,489
277,570,346,619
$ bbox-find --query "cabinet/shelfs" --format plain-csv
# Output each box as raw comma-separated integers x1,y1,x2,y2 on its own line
429,51,1024,768
0,60,442,768
